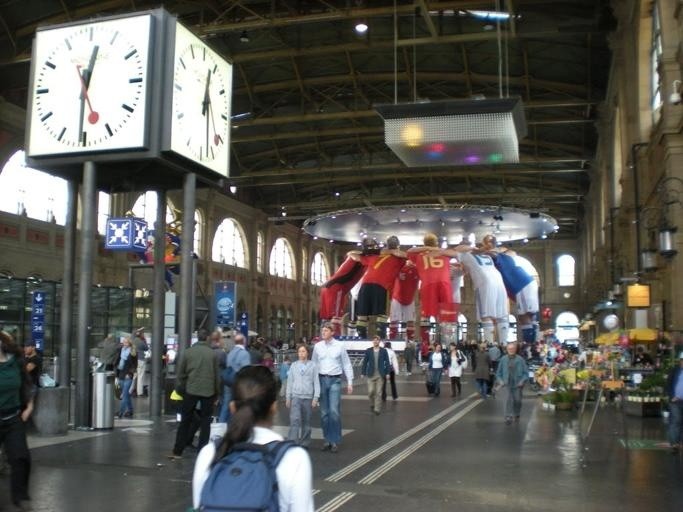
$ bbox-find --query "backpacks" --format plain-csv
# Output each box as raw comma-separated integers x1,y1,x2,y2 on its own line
198,435,299,512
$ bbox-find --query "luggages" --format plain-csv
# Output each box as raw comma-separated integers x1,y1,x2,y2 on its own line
427,369,435,394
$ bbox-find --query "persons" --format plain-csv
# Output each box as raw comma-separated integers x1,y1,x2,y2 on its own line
495,343,529,425
633,346,653,367
360,334,398,413
309,323,354,453
284,343,320,448
23,337,41,425
165,328,223,459
206,327,292,424
668,352,683,447
0,332,29,510
192,365,313,512
404,337,531,399
320,232,539,322
102,327,147,419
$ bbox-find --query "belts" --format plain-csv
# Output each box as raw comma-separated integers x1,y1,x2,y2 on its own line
319,375,339,378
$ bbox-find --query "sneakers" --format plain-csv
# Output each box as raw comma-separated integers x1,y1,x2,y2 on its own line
320,442,330,451
330,442,337,453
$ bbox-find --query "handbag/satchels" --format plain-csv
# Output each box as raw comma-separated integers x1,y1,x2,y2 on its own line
220,367,236,387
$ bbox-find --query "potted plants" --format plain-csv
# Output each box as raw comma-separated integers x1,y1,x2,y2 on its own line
542,376,574,412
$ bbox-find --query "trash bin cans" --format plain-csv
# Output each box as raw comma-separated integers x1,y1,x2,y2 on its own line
92,371,115,430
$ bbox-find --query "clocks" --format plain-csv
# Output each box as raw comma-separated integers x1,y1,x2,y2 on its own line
25,9,237,186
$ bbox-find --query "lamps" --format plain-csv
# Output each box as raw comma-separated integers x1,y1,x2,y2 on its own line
373,0,526,173
625,282,652,308
